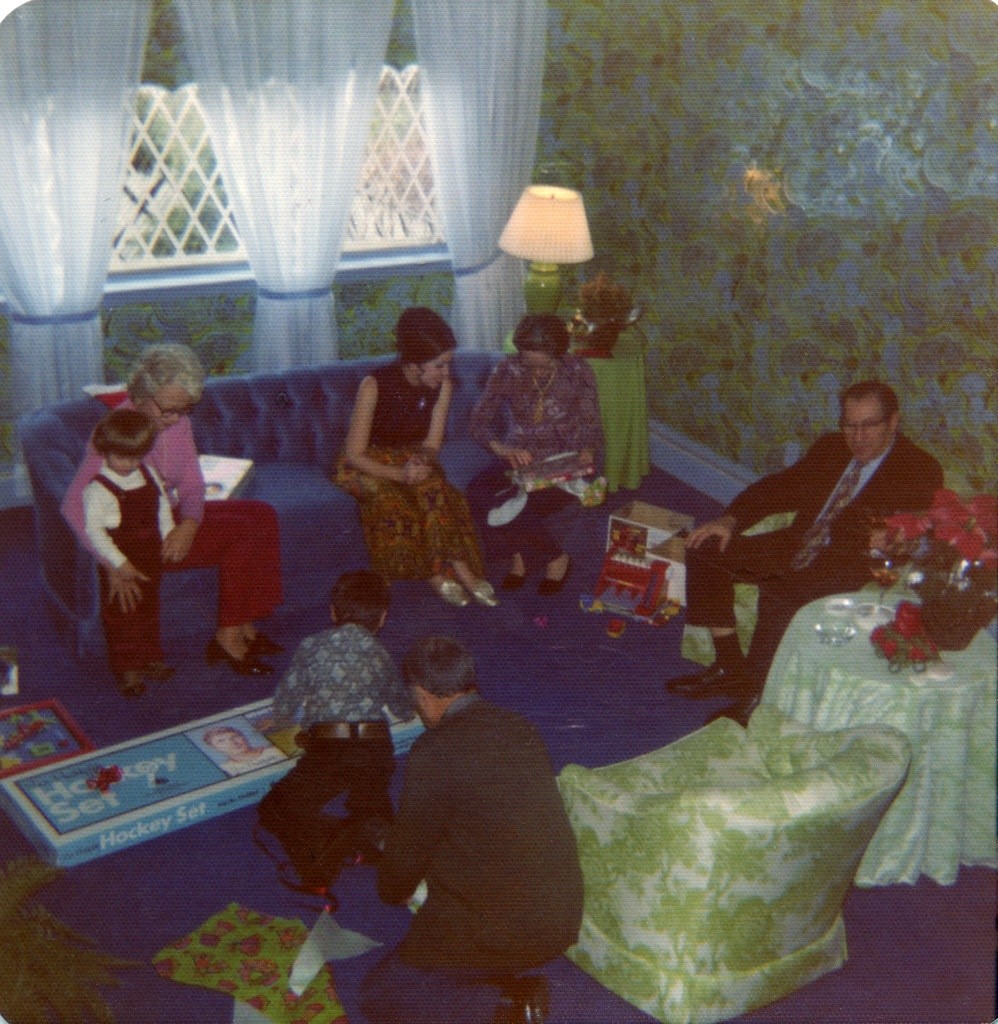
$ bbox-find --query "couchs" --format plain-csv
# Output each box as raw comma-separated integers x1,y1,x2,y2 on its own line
679,499,918,668
549,705,912,1023
16,347,501,673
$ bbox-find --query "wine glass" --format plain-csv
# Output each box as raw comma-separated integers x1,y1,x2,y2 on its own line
853,530,910,631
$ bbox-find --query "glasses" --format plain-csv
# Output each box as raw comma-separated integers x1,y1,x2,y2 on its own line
150,397,194,419
838,415,894,436
519,357,561,373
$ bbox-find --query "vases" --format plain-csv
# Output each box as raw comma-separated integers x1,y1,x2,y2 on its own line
580,311,625,355
918,554,997,655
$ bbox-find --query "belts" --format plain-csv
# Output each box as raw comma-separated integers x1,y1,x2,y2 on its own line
310,718,389,741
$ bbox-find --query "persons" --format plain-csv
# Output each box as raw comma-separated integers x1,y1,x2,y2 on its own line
205,724,288,777
331,307,503,611
261,571,398,878
472,313,607,592
62,341,284,694
358,634,583,1024
665,382,946,727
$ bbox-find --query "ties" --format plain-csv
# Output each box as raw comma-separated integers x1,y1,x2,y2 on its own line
791,460,867,573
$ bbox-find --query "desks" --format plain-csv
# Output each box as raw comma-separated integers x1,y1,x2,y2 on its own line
757,586,998,884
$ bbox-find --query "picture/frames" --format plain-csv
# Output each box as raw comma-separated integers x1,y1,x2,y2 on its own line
0,693,98,777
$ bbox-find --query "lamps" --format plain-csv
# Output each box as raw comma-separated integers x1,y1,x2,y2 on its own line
494,186,595,318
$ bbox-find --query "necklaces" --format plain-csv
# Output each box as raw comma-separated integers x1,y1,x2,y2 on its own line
413,387,430,411
160,430,172,488
529,372,555,426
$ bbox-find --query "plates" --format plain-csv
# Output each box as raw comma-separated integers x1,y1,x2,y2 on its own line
825,598,855,617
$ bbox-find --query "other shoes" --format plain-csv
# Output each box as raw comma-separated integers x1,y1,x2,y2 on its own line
428,579,473,608
536,556,571,595
145,659,177,682
497,554,529,590
491,975,552,1024
279,862,337,913
245,630,281,658
117,668,147,700
466,575,501,607
203,637,275,677
342,815,390,865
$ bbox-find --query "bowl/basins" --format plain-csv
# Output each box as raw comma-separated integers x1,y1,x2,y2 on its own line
815,620,857,645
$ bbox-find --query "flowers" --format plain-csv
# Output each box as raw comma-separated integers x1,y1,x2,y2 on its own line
572,275,641,322
885,488,998,592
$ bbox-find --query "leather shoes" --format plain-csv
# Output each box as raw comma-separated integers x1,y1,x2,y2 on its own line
705,694,766,728
665,651,748,698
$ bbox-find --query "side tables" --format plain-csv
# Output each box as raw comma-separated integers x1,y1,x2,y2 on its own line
503,315,651,490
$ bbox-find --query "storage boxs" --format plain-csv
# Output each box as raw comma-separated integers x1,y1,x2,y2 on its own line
0,686,428,869
648,536,690,607
601,499,688,587
